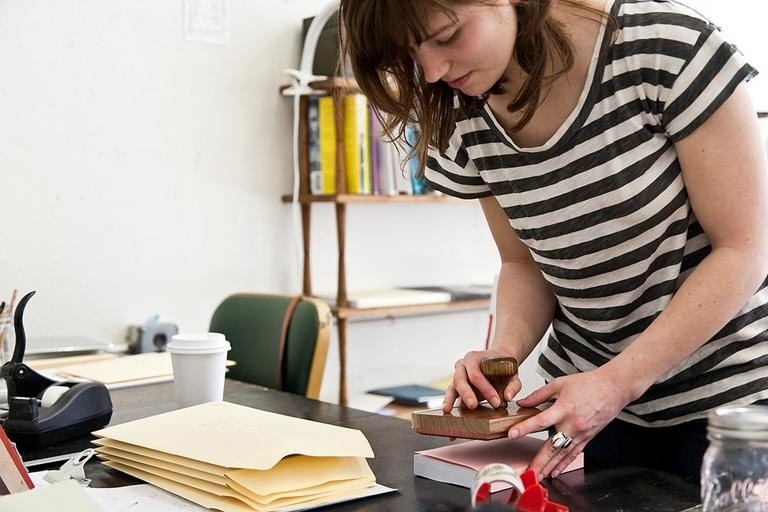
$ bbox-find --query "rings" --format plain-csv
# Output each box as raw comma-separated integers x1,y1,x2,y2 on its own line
552,431,573,451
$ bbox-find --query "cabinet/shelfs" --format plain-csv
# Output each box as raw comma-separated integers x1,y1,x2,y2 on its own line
282,76,496,433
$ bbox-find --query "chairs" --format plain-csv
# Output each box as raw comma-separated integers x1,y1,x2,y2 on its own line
207,294,330,399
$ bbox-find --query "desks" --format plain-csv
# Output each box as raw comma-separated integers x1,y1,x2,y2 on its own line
0,358,703,511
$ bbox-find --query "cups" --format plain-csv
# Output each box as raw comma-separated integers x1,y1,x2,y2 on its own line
167,332,231,409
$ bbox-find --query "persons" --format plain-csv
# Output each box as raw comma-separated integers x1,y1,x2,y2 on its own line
337,2,767,483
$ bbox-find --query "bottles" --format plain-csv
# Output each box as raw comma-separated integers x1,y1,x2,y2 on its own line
699,405,768,512
0,313,13,368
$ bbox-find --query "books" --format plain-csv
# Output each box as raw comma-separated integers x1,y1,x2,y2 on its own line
307,91,429,195
369,380,444,410
414,437,585,495
317,288,451,309
